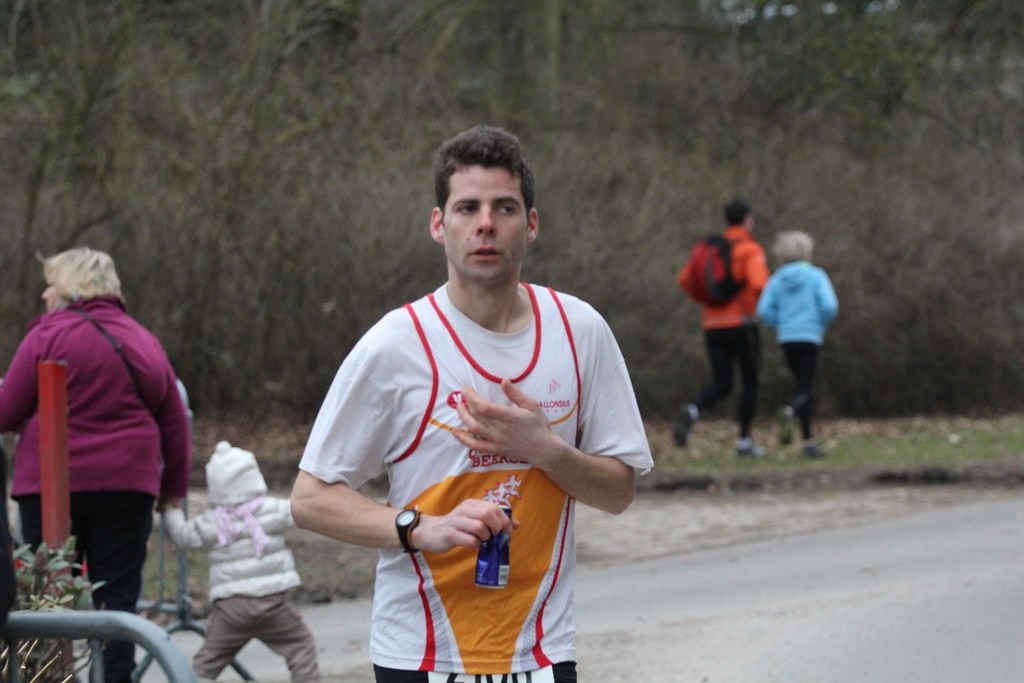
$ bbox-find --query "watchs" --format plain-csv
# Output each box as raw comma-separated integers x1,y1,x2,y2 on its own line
395,508,423,554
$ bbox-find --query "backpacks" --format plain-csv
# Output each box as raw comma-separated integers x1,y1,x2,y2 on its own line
686,236,746,309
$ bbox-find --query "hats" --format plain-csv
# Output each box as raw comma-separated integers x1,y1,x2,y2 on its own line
204,441,267,506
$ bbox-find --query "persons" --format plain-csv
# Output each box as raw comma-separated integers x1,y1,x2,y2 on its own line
760,232,838,456
161,441,322,683
290,124,654,683
672,201,772,458
0,246,191,683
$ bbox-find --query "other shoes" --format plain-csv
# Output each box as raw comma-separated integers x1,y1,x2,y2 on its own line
803,445,817,456
736,436,753,453
673,408,692,446
776,405,795,445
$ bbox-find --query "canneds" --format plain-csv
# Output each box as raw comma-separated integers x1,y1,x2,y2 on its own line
474,505,512,589
